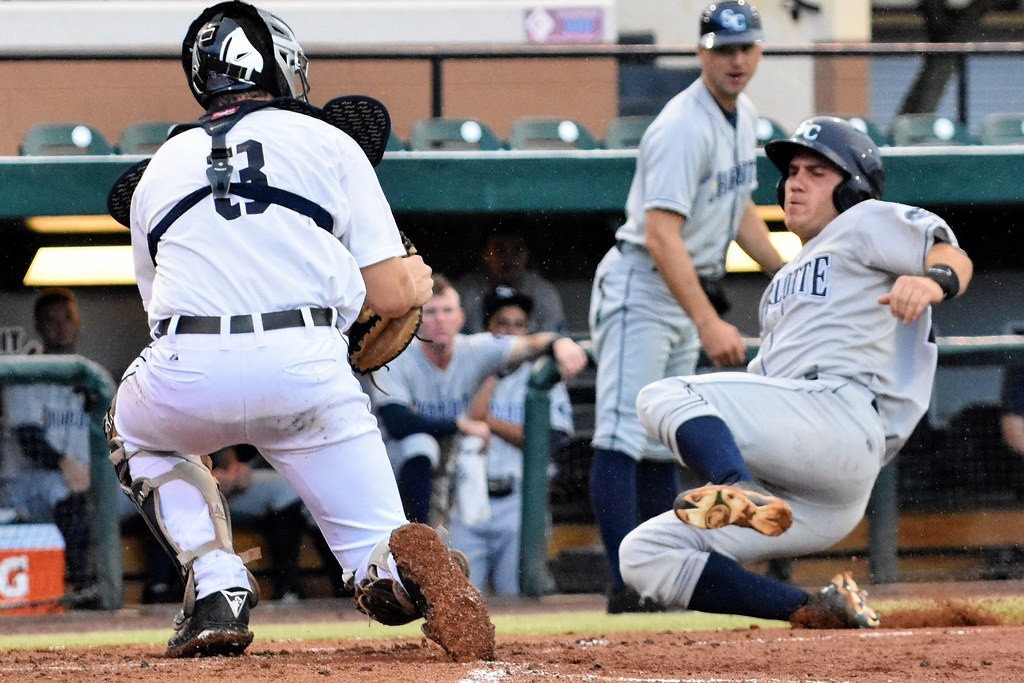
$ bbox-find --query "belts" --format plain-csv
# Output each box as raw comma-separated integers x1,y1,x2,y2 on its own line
616,239,713,286
490,484,516,499
804,372,882,416
151,306,338,338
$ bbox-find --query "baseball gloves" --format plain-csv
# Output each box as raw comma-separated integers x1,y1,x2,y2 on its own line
347,229,434,396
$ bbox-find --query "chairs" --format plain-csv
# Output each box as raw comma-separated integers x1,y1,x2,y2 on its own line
384,113,657,150
17,118,175,157
756,111,1023,150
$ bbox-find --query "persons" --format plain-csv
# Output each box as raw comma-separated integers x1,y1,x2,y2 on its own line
118,441,309,605
588,0,787,616
358,271,589,529
0,288,184,610
106,0,495,661
455,221,570,336
616,114,974,628
443,280,573,597
997,355,1023,507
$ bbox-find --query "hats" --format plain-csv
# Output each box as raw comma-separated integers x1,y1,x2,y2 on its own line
480,281,536,327
699,0,767,50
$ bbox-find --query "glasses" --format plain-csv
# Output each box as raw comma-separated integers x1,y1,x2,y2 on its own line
421,305,462,318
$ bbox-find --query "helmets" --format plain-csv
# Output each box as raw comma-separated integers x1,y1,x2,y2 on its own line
181,0,312,104
765,116,887,213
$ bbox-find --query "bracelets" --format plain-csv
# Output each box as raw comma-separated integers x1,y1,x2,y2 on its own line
923,263,961,301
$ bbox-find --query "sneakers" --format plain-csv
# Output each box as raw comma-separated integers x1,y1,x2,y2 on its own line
388,522,497,665
805,568,882,629
164,554,256,657
605,583,664,614
673,470,794,536
